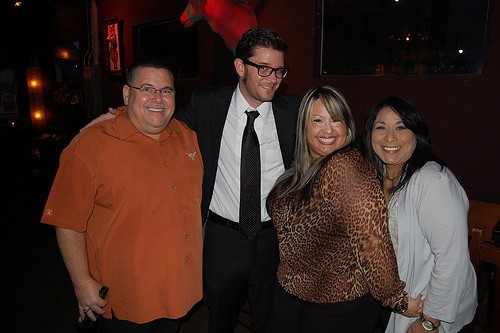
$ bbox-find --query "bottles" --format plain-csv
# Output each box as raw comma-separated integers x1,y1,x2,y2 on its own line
74,286,109,330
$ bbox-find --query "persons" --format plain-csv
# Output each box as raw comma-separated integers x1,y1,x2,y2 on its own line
40,60,210,331
362,93,479,333
82,27,303,333
265,84,426,333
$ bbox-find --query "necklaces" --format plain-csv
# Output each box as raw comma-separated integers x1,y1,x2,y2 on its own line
386,171,401,180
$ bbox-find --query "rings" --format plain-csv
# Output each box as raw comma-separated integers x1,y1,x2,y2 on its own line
83,306,90,312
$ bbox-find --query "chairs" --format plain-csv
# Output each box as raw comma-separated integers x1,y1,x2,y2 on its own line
463,226,500,333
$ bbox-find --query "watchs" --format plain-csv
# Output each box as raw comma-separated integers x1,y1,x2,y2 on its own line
391,295,409,314
419,312,441,331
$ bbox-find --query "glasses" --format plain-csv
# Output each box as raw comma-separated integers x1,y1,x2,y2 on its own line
242,59,288,79
125,83,176,100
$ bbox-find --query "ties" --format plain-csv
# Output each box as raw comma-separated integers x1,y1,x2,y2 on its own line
238,110,261,241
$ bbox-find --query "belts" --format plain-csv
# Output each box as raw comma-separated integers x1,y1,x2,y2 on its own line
209,211,275,231
